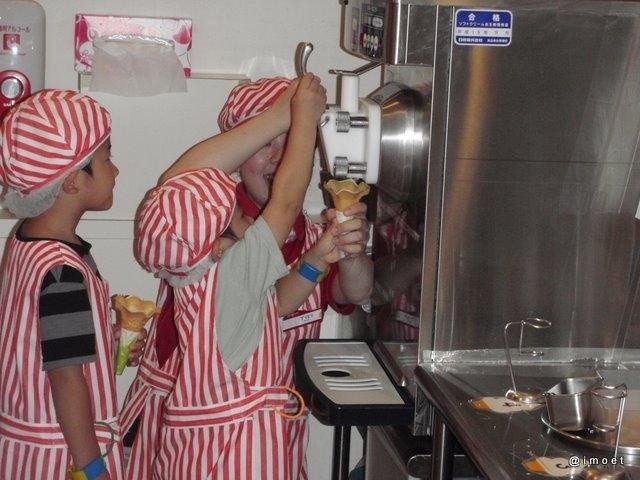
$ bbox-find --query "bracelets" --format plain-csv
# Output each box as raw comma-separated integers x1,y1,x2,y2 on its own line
69,455,107,479
296,253,331,284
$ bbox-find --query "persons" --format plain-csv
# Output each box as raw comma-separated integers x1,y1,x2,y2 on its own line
117,75,374,479
130,73,328,479
1,88,150,480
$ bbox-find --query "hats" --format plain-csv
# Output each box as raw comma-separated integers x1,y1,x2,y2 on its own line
134,169,237,274
0,88,111,194
217,78,292,133
